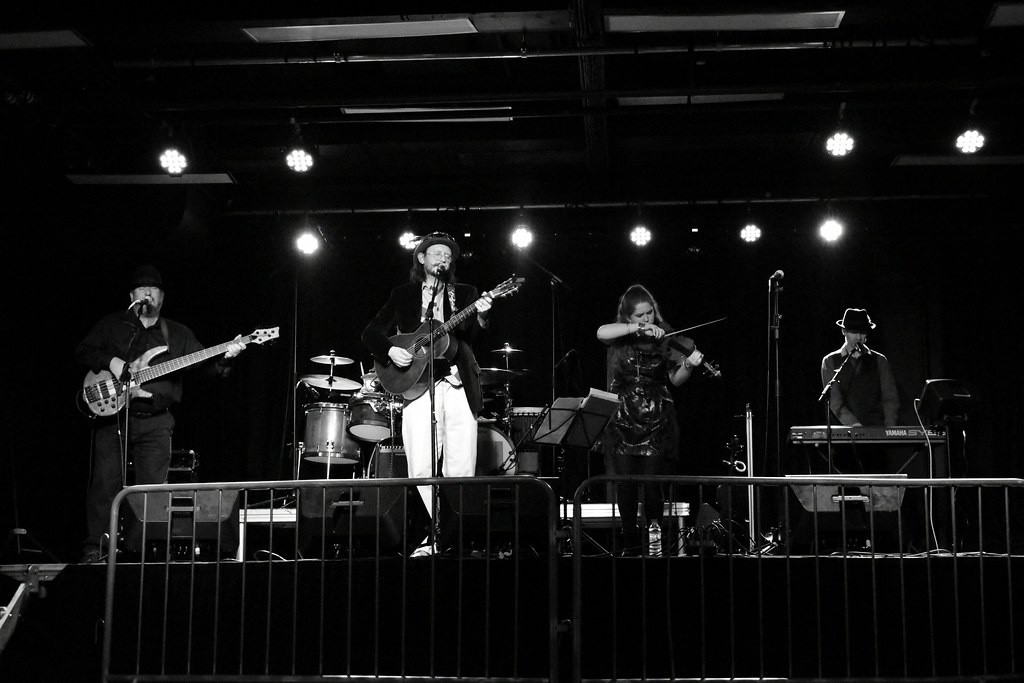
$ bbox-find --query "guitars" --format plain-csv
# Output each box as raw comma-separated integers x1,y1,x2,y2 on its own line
377,273,527,401
81,326,280,417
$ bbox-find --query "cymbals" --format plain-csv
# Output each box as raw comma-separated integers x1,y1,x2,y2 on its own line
299,374,363,390
508,368,530,375
492,349,522,354
480,368,531,375
309,355,354,366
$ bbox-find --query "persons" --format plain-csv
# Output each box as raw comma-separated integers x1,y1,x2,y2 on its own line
596,284,704,556
821,307,901,550
74,267,247,564
361,230,495,557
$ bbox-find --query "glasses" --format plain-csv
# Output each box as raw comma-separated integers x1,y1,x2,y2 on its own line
424,232,456,242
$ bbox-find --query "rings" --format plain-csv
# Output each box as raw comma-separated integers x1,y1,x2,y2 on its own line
480,303,483,307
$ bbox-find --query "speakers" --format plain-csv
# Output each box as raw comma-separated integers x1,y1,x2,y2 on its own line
123,487,241,560
295,484,551,558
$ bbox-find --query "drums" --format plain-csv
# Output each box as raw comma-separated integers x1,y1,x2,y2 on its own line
374,444,408,478
345,391,397,442
474,423,519,476
301,402,361,464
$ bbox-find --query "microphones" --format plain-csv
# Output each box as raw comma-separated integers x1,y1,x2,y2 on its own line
135,296,152,305
439,264,448,272
856,341,865,354
489,469,501,477
770,270,784,280
301,380,320,399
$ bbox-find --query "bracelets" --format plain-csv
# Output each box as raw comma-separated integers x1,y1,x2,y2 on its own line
684,358,694,372
638,322,645,336
627,324,630,334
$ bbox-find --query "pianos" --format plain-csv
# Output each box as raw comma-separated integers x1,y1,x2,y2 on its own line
788,423,966,553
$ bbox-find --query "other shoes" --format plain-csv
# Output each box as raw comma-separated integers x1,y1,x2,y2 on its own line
411,535,437,557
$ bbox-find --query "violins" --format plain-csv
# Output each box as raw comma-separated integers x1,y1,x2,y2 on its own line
636,323,722,378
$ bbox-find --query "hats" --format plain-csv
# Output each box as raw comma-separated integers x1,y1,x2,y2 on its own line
834,306,877,333
413,231,460,268
129,263,166,293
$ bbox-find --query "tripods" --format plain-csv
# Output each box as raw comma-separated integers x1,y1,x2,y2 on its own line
531,405,614,558
